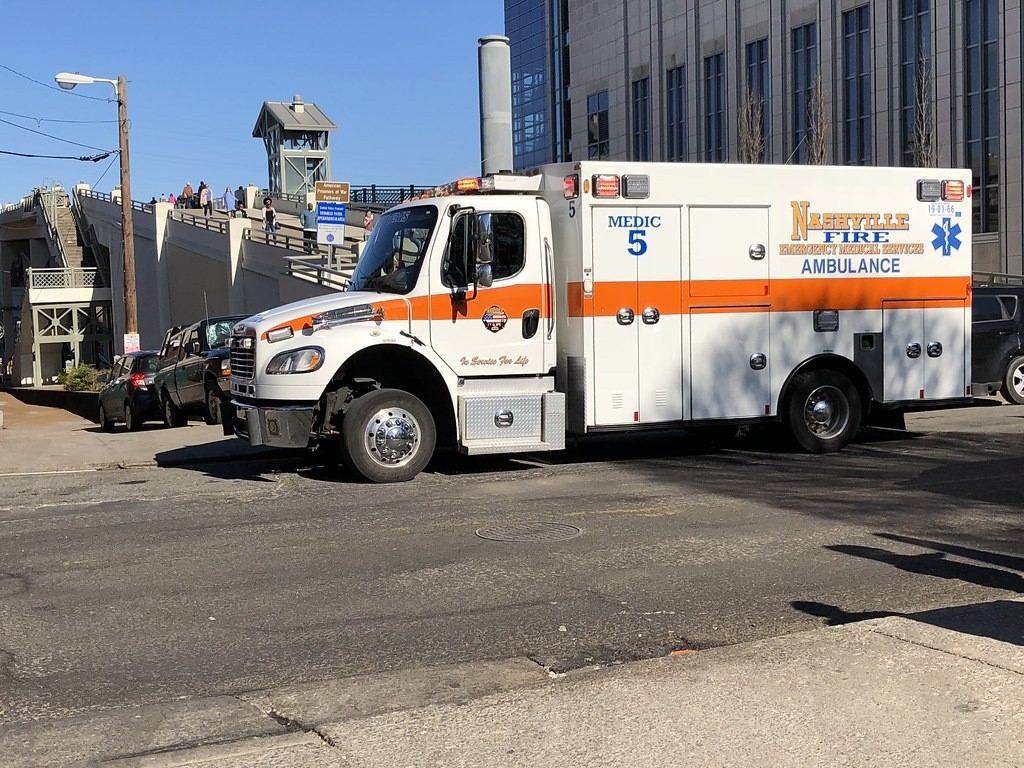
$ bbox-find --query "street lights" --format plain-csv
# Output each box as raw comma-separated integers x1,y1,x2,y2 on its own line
54,71,141,353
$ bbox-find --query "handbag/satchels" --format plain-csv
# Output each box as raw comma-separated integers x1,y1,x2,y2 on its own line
183,193,187,198
275,222,280,229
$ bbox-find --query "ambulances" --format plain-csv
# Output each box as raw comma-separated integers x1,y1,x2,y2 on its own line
230,157,972,483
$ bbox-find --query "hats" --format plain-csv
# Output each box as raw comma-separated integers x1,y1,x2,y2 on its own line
265,200,271,205
186,182,190,186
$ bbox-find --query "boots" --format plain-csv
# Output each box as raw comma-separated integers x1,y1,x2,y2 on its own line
273,235,278,244
266,237,270,245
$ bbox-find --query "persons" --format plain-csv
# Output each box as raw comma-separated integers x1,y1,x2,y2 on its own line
160,193,166,202
300,203,320,254
363,209,374,240
261,198,276,245
149,197,157,209
231,201,247,218
407,228,429,253
222,187,238,218
200,185,213,219
393,230,401,264
198,181,206,208
168,193,177,208
182,182,193,209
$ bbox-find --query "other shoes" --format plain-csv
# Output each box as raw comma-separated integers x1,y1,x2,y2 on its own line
313,248,320,254
210,217,214,220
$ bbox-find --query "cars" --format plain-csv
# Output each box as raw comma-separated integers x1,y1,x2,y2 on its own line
970,286,1024,405
154,314,253,428
99,350,160,432
